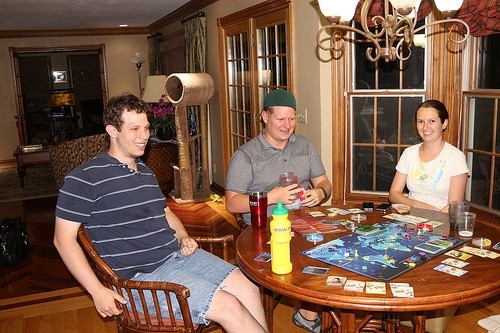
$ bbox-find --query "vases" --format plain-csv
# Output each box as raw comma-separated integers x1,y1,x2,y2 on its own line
155,126,173,141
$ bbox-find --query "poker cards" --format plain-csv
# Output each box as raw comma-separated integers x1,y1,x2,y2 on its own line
302,265,330,276
325,275,415,298
307,210,325,217
326,207,365,216
382,212,444,229
432,245,500,277
254,252,271,263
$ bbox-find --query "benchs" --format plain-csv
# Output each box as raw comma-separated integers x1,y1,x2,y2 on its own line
49,132,179,196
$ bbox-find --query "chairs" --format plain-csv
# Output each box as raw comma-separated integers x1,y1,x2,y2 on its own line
77,224,235,333
232,212,250,230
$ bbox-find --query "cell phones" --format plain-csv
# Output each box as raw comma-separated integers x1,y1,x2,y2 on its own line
376,203,392,211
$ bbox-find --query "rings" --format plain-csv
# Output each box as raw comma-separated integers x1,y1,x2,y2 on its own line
313,199,317,203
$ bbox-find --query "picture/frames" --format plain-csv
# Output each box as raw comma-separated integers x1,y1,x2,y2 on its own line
52,70,68,84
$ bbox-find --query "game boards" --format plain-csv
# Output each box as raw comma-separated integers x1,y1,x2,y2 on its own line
298,219,467,282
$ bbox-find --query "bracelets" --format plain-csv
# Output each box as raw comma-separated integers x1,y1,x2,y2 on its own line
316,187,327,200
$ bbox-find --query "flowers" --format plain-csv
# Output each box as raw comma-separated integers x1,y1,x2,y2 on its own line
145,94,175,127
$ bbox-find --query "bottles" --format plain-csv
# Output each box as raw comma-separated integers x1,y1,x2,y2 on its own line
270,202,293,275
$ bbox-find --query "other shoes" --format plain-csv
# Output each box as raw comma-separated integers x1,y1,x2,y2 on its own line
292,308,321,333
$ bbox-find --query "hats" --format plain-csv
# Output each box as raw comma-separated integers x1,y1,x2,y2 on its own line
264,89,296,110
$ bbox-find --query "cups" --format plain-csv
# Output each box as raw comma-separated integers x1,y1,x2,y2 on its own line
449,201,471,231
457,212,476,237
249,192,268,230
279,172,296,188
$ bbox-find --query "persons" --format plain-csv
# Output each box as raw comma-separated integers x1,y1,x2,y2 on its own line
226,89,332,333
53,95,268,333
389,100,470,213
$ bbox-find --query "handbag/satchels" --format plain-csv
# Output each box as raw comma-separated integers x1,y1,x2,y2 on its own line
0,217,32,266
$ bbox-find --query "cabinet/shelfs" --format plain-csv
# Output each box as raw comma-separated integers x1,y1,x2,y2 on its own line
48,89,81,142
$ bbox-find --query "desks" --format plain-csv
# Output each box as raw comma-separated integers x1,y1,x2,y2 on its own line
234,203,500,333
14,145,51,189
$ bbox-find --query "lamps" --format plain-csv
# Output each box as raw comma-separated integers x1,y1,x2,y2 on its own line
141,71,171,103
128,51,148,100
311,0,470,62
